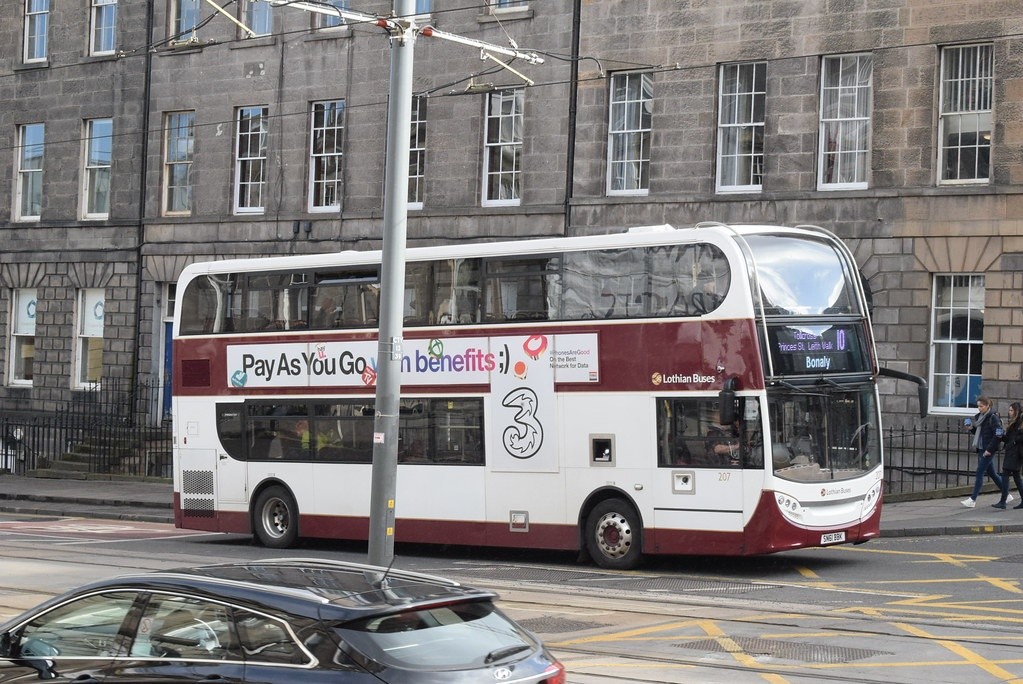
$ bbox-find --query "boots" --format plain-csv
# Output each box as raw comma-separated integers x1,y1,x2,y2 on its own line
991,493,1009,509
1013,491,1023,509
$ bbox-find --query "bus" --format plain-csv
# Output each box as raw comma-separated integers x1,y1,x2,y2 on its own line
168,219,892,571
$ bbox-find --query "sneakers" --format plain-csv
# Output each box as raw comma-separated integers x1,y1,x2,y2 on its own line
1006,494,1014,503
960,497,975,507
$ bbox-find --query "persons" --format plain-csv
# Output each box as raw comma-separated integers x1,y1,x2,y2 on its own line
293,416,343,459
315,298,337,329
689,269,726,316
991,402,1023,510
436,281,471,324
960,395,1013,508
705,411,751,465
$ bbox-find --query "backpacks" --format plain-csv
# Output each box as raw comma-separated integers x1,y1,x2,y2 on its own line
990,414,1005,451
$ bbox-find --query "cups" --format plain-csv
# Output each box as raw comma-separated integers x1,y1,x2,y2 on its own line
964,418,971,426
996,428,1002,436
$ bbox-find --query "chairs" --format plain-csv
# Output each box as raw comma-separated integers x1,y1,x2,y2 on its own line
243,312,547,329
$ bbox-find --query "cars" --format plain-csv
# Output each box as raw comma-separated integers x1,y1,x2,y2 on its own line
0,556,567,684
